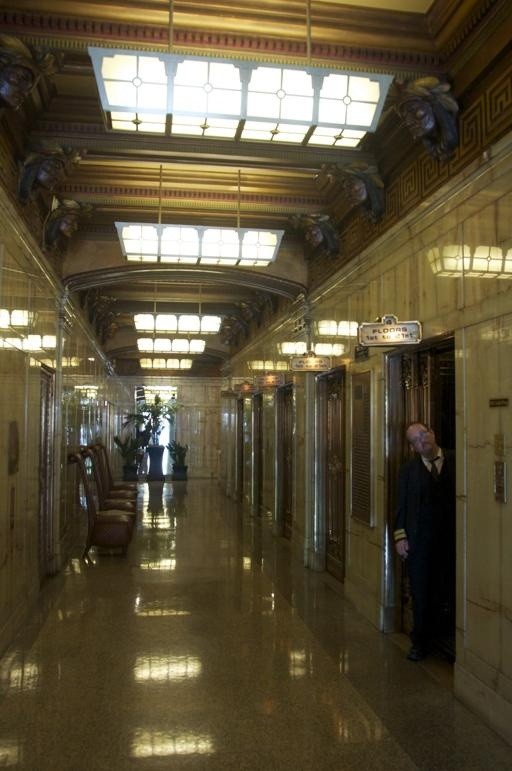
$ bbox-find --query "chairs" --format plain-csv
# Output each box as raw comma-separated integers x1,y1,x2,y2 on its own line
66,442,140,562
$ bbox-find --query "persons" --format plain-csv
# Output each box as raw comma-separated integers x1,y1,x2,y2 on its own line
0,31,44,114
394,76,459,160
337,161,387,224
42,199,86,249
79,286,117,346
220,289,277,344
392,421,455,661
293,215,342,258
19,141,70,193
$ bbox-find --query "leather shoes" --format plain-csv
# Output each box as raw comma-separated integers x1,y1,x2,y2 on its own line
406,642,422,662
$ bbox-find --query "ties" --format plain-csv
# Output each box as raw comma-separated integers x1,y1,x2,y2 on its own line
430,462,439,480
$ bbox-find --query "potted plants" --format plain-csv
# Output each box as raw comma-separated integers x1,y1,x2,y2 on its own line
112,391,189,483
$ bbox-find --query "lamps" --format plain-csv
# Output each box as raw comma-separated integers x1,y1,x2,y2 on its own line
426,205,512,283
0,285,83,374
